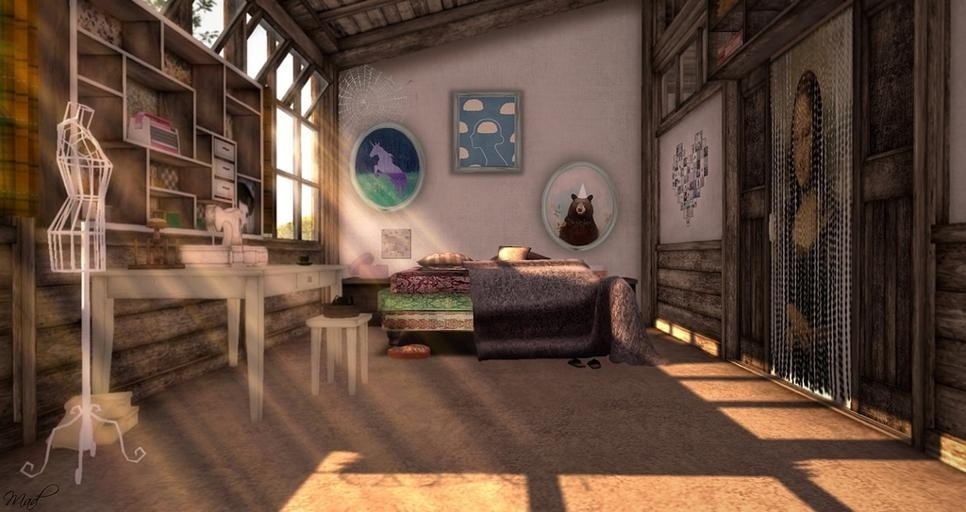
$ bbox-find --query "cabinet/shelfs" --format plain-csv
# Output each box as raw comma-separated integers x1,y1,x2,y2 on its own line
42,1,273,242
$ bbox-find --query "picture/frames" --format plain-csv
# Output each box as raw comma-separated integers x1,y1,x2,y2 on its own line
450,87,525,176
540,161,620,255
350,123,425,213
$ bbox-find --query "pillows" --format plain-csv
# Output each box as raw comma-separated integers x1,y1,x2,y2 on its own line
416,245,551,268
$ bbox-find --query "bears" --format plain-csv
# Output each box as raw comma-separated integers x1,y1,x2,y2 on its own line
559,193,599,246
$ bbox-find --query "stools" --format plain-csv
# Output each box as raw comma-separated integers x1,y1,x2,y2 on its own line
306,313,373,396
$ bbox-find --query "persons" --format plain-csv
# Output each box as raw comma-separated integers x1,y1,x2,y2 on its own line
772,68,843,396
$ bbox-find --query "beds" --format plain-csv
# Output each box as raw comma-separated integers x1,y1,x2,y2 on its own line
383,259,596,347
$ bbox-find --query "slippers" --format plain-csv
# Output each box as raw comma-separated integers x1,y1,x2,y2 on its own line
568,358,586,367
588,359,602,369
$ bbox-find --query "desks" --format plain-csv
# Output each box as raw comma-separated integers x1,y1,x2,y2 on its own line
92,264,345,424
